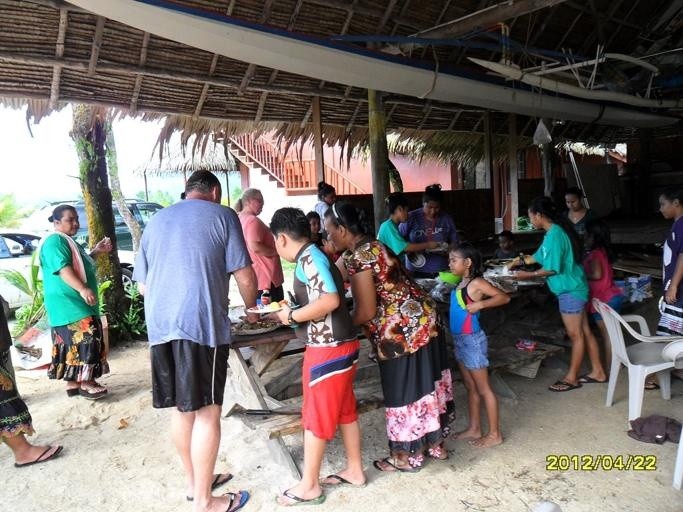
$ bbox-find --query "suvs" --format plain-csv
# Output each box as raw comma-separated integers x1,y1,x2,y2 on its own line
72,199,168,251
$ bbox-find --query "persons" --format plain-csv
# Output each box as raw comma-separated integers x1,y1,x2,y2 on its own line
399,183,458,280
236,187,285,303
131,170,260,512
1,304,64,467
644,191,682,390
324,204,455,473
564,188,595,239
38,205,112,399
446,243,511,448
270,208,368,506
376,193,438,261
307,181,343,263
495,229,519,258
511,198,607,392
581,221,624,372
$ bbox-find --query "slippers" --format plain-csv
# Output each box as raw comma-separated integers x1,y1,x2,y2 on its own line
188,472,250,512
577,373,608,384
549,381,582,392
68,383,107,400
375,456,421,472
14,446,63,468
275,484,325,506
321,474,367,489
645,381,659,390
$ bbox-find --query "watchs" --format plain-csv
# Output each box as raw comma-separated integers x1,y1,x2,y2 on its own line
288,311,299,325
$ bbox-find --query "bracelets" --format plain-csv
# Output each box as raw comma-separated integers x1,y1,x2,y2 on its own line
535,271,539,278
519,257,526,265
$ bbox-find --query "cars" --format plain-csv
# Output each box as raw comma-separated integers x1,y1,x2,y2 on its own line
0,227,135,320
23,198,78,231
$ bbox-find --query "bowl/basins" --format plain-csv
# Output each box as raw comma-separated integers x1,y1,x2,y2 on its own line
413,278,437,294
485,259,515,269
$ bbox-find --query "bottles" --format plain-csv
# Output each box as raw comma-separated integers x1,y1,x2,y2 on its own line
518,252,525,267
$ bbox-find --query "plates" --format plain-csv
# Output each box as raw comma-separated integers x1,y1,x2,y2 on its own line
247,302,299,313
230,322,282,336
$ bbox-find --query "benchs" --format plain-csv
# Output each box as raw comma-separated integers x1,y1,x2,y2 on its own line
241,342,565,481
226,334,365,401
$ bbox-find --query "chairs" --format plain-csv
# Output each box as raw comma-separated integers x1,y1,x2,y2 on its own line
592,297,683,430
